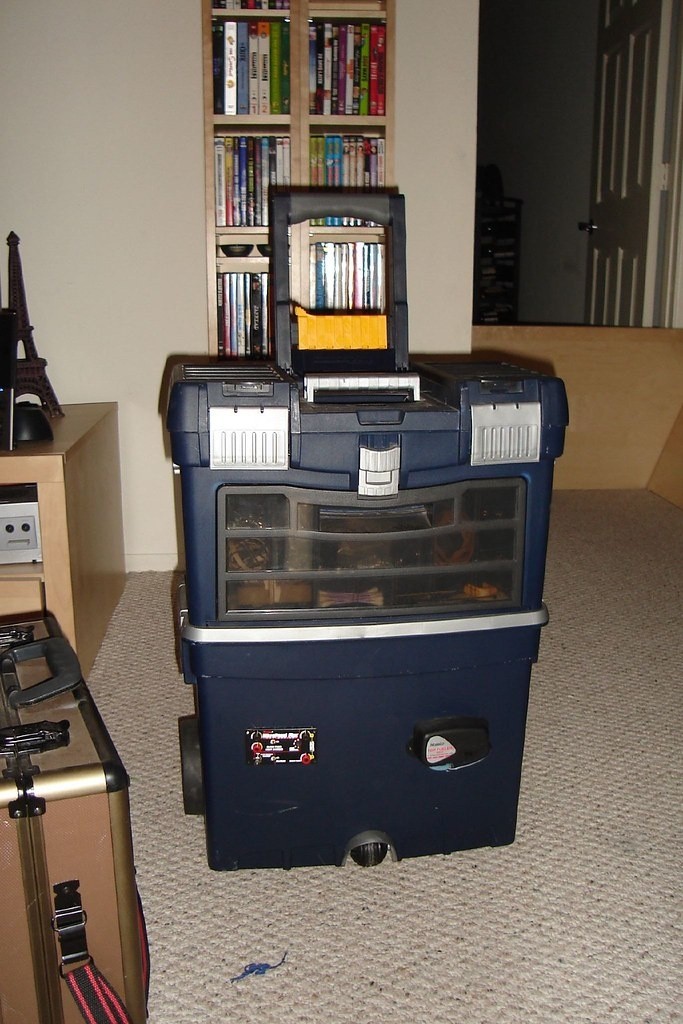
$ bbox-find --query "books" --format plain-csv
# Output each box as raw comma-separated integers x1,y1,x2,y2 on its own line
213,1,288,362
309,15,389,313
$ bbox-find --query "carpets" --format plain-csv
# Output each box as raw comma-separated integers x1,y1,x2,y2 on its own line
87,487,683,1024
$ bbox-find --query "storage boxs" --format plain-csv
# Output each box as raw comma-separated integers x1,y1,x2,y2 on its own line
1,609,154,1023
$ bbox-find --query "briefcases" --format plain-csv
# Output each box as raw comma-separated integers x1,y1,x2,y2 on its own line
0,611,152,1022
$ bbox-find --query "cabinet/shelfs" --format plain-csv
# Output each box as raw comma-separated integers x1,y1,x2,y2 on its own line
472,164,524,325
1,401,127,692
199,1,397,359
